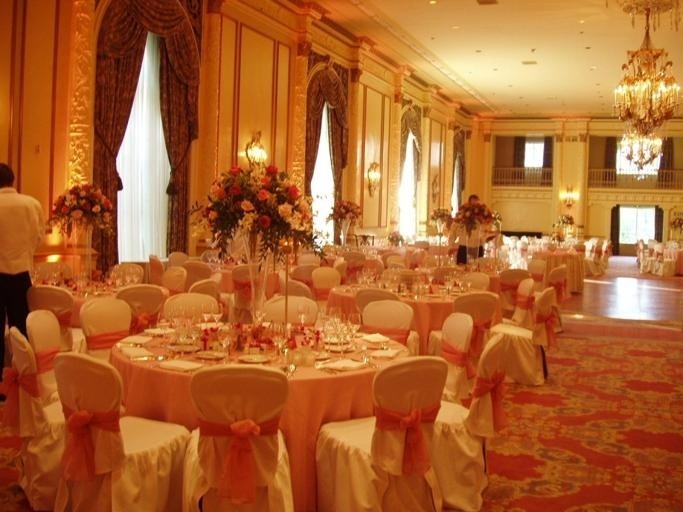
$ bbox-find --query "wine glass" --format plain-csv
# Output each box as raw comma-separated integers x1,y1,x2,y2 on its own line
50,272,59,287
112,264,143,289
76,271,88,297
213,251,219,270
207,250,212,266
345,264,472,301
157,302,363,364
95,271,113,294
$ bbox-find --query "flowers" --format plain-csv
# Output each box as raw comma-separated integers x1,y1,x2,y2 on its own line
44,182,114,237
185,164,329,271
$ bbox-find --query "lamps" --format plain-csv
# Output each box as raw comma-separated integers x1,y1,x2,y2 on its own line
367,162,382,196
613,0,683,169
246,130,268,170
559,185,580,207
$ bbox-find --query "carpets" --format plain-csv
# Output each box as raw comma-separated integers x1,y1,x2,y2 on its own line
0,319,682,512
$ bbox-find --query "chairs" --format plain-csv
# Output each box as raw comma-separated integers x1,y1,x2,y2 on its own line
54,351,190,512
10,327,66,511
181,364,294,512
316,356,448,512
635,218,683,275
431,332,504,512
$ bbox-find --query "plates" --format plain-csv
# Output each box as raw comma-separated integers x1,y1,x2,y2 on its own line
195,349,226,361
237,355,270,364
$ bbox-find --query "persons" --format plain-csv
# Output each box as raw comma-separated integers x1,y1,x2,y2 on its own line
447,194,486,265
0,162,46,401
